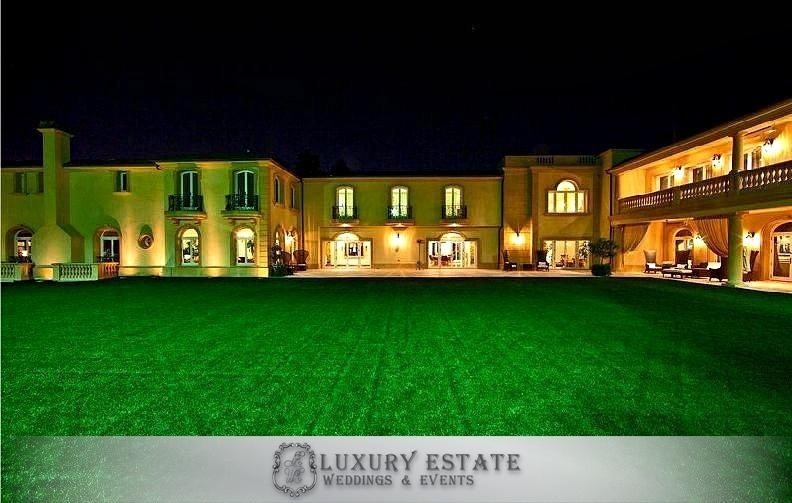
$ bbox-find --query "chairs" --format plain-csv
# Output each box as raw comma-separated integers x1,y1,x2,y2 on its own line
429,254,452,266
503,250,517,272
536,250,549,272
643,249,759,282
282,251,295,275
293,250,309,271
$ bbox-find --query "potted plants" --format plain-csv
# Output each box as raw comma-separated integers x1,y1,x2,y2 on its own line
588,238,618,276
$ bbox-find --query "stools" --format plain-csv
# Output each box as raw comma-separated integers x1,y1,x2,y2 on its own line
522,263,532,271
